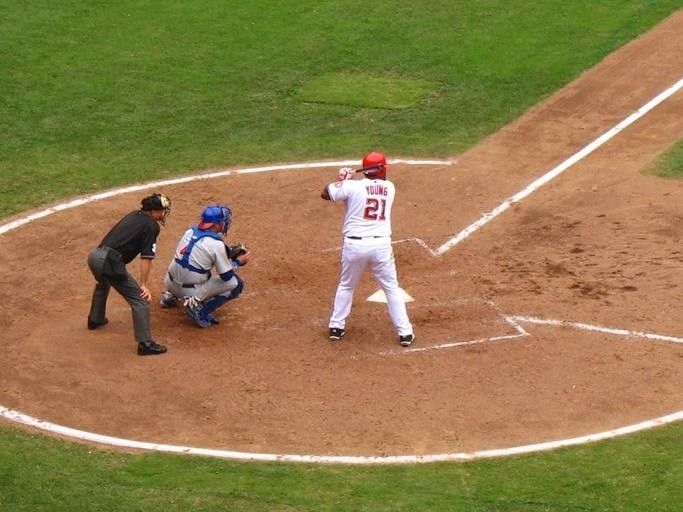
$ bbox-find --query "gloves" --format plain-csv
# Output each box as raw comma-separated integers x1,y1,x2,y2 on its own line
338,167,355,181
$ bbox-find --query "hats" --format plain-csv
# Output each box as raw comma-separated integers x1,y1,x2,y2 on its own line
197,205,231,232
141,193,170,212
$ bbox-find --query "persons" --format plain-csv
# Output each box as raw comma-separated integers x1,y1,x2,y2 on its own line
320,151,414,345
161,204,251,328
87,194,171,355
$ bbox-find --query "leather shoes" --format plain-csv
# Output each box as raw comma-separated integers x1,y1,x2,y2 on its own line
87,316,109,330
137,341,167,355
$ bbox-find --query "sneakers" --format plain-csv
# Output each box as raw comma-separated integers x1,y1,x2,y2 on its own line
328,327,346,341
160,290,183,309
185,307,213,329
399,332,416,346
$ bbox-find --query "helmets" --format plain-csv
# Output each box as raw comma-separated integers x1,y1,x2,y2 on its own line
361,152,389,180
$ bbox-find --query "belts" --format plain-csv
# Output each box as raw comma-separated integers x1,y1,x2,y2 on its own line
168,271,194,289
347,236,381,239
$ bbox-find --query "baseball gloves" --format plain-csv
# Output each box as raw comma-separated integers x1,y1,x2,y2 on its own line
228,241,249,266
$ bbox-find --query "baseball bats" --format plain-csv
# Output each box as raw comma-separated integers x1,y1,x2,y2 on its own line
349,164,384,172
